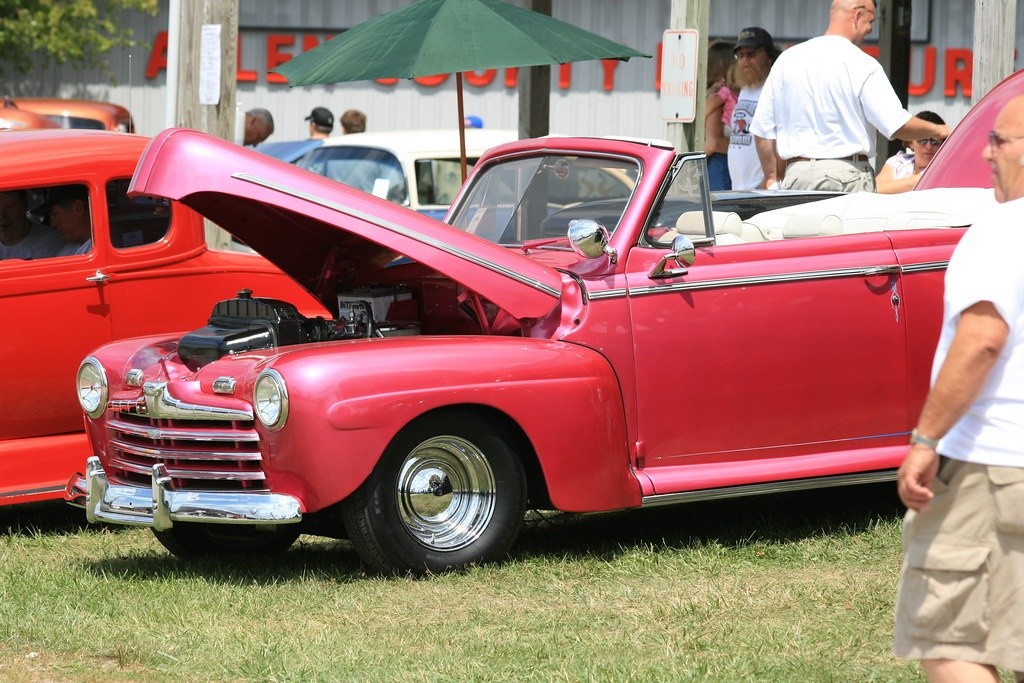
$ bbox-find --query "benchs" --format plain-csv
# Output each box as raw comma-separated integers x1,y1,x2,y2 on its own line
750,186,998,240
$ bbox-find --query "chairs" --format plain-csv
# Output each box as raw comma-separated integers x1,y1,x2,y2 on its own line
785,214,841,238
668,212,749,252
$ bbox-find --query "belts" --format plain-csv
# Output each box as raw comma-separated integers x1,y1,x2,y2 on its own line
787,154,870,163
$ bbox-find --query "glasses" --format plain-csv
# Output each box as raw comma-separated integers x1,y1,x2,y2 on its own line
916,138,940,146
989,130,1024,149
734,50,761,60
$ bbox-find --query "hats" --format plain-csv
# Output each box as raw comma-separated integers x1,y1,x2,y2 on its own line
732,27,774,51
30,185,85,216
304,107,334,128
464,115,483,128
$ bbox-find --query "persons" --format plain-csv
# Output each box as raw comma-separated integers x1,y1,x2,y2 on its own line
431,116,483,204
235,108,274,148
0,185,91,258
705,0,952,191
893,95,1024,683
297,107,407,204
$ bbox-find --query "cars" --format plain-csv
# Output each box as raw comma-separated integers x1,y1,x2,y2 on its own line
0,94,135,134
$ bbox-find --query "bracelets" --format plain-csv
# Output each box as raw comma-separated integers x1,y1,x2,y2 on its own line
910,429,938,447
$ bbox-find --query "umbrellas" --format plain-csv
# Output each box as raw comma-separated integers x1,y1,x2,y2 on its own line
267,0,653,185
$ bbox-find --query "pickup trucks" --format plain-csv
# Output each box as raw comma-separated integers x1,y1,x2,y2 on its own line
63,68,1023,559
219,132,852,248
0,125,342,509
